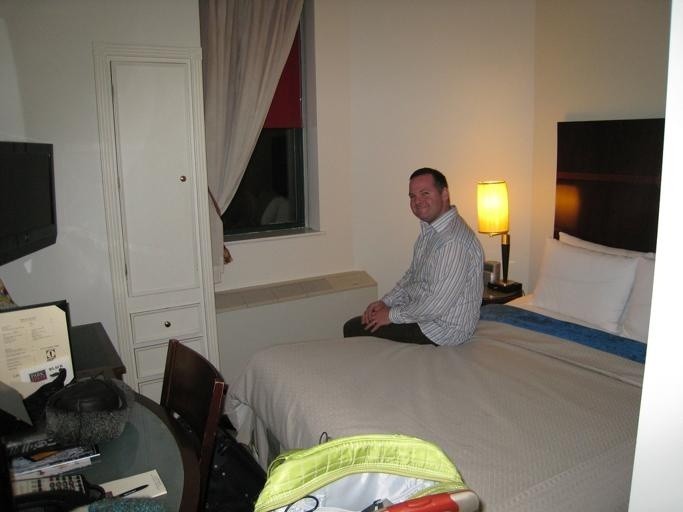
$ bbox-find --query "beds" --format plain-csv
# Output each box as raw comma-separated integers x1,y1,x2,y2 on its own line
224,292,645,511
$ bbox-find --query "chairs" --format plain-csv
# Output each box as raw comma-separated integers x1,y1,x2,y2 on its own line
146,337,228,511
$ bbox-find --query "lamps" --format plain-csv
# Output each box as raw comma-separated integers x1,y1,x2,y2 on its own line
474,175,522,294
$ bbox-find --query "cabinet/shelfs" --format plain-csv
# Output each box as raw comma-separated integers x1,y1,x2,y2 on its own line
88,40,219,404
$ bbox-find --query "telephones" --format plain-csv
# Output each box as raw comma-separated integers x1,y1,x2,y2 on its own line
11,473,106,512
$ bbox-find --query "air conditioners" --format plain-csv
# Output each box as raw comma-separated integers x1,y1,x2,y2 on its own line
213,269,380,358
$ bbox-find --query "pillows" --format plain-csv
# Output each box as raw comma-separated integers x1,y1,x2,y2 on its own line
529,233,639,331
555,230,653,345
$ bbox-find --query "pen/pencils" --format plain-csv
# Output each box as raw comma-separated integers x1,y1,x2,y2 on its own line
111,484,149,501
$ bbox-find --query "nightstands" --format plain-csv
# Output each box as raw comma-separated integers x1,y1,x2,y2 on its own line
480,289,522,308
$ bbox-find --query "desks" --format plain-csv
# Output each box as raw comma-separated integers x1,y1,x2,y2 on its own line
68,319,126,382
59,388,203,512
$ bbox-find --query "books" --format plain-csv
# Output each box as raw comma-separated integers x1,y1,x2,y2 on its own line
8,430,102,478
98,468,169,501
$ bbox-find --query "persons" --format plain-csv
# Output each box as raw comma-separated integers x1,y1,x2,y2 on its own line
342,165,487,347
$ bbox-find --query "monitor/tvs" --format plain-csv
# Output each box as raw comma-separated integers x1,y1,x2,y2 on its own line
0,141,57,267
